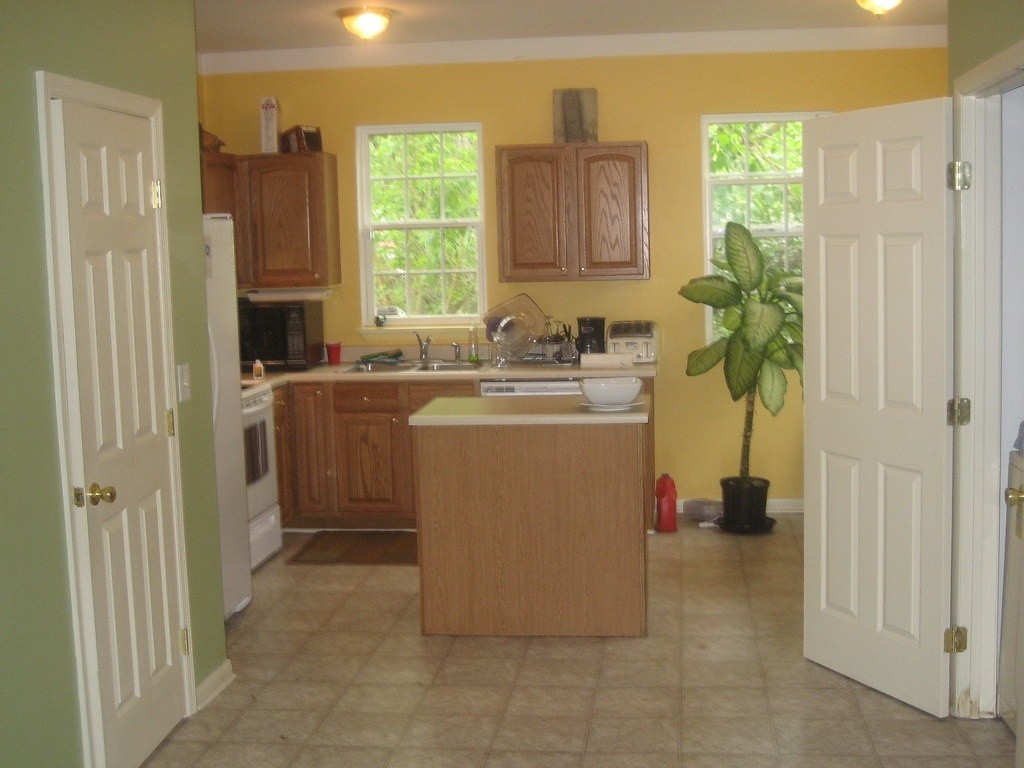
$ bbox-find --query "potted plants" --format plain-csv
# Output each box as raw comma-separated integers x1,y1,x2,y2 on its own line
679,222,803,537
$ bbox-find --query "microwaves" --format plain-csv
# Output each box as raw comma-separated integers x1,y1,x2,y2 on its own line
238,296,325,373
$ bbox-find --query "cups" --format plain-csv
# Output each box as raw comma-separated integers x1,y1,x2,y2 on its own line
326,341,342,366
488,343,508,368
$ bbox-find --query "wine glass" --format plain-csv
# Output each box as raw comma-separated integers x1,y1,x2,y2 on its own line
542,315,553,342
554,320,563,342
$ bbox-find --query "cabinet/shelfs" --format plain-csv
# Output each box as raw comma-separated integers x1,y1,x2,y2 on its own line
496,138,650,284
292,383,336,518
272,385,295,525
200,150,247,290
334,383,415,530
409,381,473,412
244,151,341,290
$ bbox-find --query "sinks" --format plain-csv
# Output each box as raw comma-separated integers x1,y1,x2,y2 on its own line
335,361,420,373
412,359,489,372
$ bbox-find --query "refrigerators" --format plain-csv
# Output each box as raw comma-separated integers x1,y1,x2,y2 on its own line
202,212,252,621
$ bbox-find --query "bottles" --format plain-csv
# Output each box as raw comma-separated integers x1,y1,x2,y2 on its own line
469,318,478,361
253,359,264,379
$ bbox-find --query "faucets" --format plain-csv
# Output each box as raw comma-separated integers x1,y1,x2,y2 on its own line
451,341,461,363
412,330,433,359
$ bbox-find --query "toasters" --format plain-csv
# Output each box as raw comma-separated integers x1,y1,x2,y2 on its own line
607,321,658,364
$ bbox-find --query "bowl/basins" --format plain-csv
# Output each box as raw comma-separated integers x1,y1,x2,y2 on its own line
578,376,644,404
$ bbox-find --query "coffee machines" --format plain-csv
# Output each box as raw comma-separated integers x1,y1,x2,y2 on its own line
575,317,605,364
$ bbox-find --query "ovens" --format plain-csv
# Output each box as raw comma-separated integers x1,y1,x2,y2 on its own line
240,379,283,569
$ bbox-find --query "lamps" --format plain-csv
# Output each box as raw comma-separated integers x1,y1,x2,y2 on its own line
336,6,395,40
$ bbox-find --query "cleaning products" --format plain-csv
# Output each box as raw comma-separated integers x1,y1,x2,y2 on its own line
467,318,479,363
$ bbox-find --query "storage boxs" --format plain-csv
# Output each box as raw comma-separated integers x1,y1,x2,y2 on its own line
260,97,279,154
282,124,322,151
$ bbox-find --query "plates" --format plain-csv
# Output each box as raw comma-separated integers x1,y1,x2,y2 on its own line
580,399,645,411
